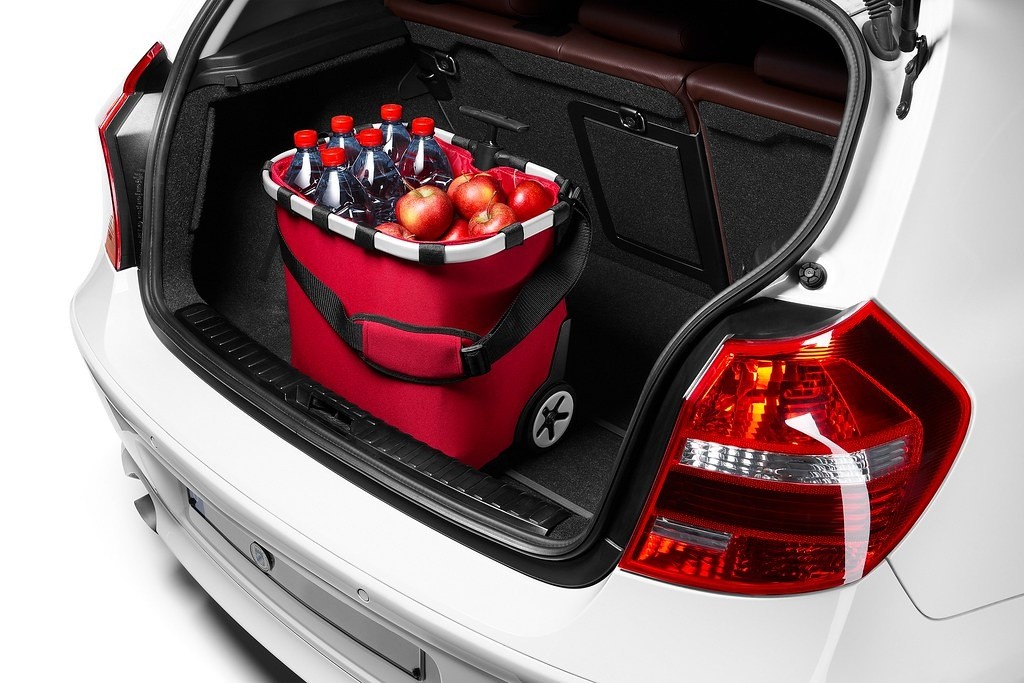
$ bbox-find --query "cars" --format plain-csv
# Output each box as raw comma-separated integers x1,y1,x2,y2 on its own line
73,0,1023,681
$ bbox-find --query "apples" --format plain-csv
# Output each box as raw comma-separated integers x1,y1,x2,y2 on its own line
374,173,553,242
